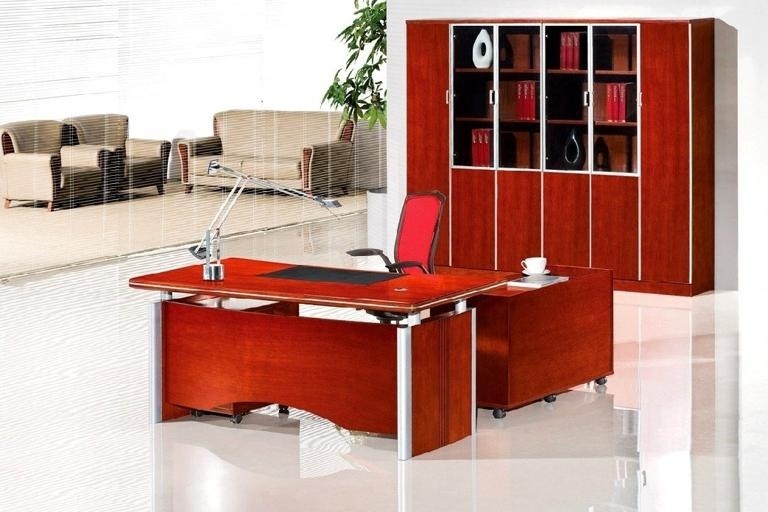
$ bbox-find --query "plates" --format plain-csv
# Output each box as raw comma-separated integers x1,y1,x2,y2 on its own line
522,269,551,276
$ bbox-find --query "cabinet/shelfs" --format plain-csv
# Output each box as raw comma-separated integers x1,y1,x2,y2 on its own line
405,19,715,297
429,265,614,418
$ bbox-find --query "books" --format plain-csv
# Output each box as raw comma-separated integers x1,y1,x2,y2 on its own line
515,80,540,120
471,127,494,168
507,274,570,289
607,82,635,124
560,32,587,71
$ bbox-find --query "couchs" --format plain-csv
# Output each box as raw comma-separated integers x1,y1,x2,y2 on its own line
177,110,355,198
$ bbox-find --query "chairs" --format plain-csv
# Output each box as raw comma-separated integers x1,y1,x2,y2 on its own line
0,120,111,212
346,189,447,326
62,114,171,202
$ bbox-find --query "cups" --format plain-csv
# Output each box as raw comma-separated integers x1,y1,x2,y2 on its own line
521,256,547,273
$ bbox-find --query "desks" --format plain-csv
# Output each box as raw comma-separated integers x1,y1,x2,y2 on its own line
129,258,528,461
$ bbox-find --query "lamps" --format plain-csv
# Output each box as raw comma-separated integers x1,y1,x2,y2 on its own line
188,159,343,281
